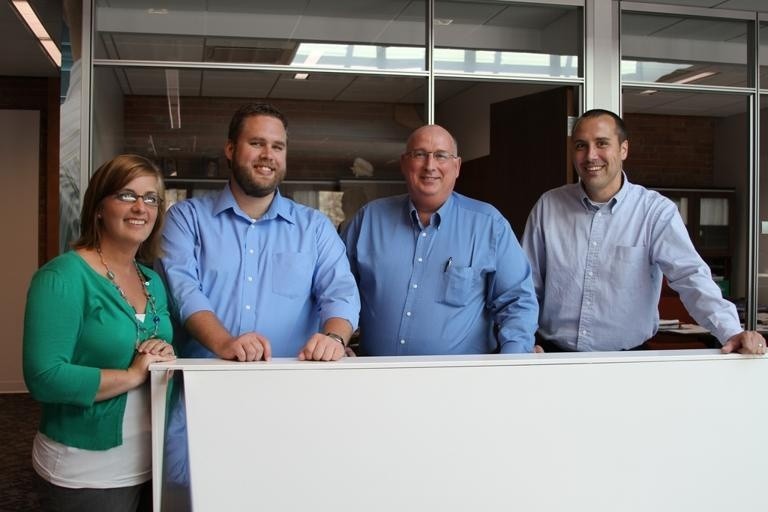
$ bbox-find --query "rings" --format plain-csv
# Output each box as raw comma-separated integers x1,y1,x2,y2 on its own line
758,344,764,348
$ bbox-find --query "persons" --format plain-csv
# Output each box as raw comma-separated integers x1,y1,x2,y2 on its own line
522,108,768,355
149,100,363,362
21,153,174,510
340,124,540,352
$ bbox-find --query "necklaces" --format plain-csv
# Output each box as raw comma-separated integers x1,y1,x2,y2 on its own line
96,237,160,350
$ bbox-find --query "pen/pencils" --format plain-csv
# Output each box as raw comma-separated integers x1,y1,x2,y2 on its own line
445,257,452,273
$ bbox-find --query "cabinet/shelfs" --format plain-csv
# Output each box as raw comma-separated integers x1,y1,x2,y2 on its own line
644,188,736,295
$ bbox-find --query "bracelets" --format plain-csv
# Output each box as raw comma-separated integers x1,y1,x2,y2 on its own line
326,331,346,348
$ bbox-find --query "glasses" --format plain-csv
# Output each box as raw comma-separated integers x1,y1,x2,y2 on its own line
117,191,163,206
410,150,456,161
